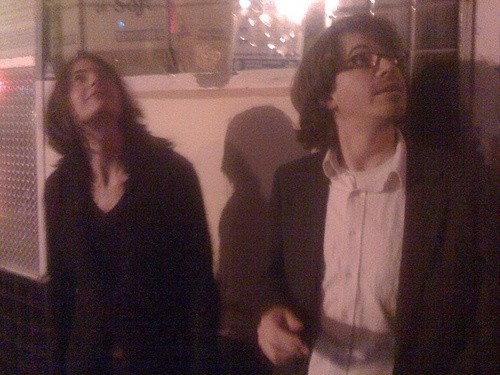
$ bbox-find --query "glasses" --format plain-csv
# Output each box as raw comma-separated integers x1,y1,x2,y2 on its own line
335,44,408,72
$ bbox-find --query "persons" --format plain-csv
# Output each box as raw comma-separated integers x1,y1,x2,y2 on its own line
41,51,214,374
255,11,500,374
